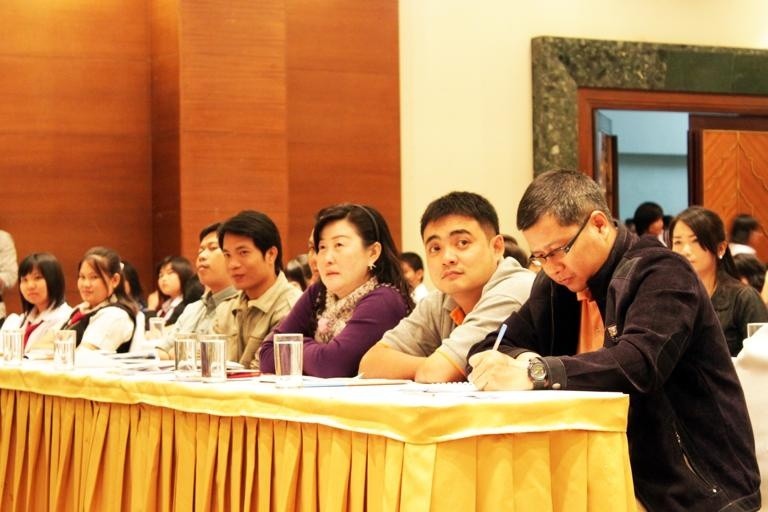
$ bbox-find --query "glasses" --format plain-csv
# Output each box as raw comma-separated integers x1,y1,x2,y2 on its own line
526,210,593,268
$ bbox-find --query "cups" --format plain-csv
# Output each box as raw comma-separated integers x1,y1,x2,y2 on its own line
174,335,200,381
200,336,226,381
53,329,77,370
746,323,765,339
0,326,24,364
148,315,165,343
273,332,305,389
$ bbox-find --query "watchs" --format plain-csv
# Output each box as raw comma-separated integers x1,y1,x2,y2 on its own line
527,359,547,391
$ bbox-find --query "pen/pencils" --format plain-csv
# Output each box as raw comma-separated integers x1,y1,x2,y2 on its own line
492,323,508,351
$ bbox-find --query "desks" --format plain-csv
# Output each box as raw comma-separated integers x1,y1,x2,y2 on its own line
0,359,650,512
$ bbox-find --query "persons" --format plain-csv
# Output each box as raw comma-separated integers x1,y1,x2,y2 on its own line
463,166,768,512
634,202,768,357
0,192,543,383
